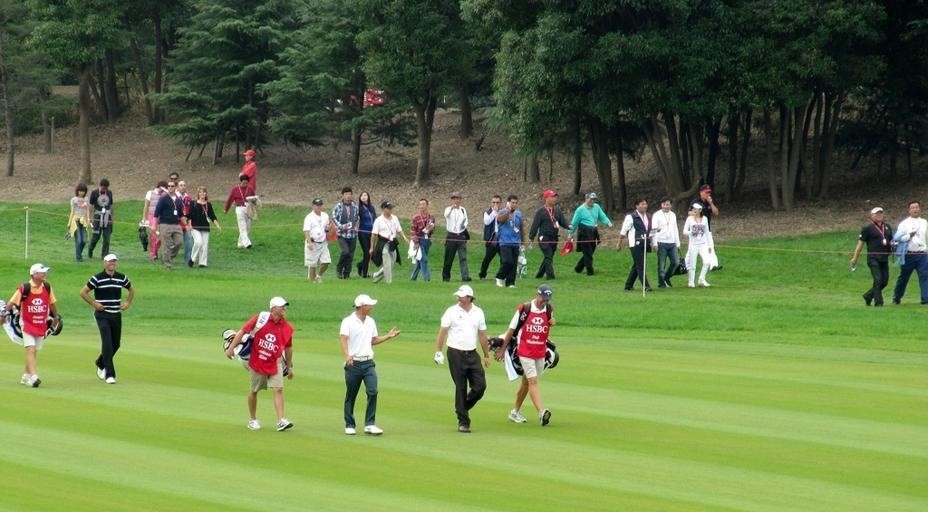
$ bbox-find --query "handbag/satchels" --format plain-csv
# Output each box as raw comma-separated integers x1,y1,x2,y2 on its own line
517,249,527,275
139,227,148,251
674,248,686,274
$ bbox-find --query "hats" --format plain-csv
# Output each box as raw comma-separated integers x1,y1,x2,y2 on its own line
380,201,395,209
451,192,461,199
453,285,472,298
584,192,598,199
29,263,50,276
692,203,702,212
269,297,289,309
870,207,883,215
561,242,573,256
543,190,558,199
312,199,323,205
535,284,552,301
355,295,376,306
699,184,711,193
243,150,256,156
103,253,118,262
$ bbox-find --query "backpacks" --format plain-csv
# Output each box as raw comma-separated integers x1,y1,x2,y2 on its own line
221,312,288,377
488,302,559,375
0,281,62,345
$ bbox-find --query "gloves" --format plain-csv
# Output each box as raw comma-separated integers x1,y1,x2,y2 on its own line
434,352,444,365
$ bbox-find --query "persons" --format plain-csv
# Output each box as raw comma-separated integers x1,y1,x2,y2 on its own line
302,187,614,289
140,173,222,269
489,282,555,425
335,293,402,436
239,150,257,193
616,185,721,291
77,254,135,384
225,295,297,433
68,183,90,261
1,263,63,389
429,282,493,433
224,174,256,250
888,201,928,304
87,179,114,260
849,208,895,308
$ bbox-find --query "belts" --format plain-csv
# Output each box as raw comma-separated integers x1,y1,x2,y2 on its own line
447,347,476,355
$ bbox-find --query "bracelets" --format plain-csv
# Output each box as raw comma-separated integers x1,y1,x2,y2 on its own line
287,367,292,370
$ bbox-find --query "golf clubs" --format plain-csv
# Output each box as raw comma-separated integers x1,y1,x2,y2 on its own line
0,299,21,320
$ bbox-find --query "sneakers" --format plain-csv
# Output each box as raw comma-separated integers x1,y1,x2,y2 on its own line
364,425,382,434
105,377,115,384
247,420,260,430
20,374,31,386
538,410,551,426
495,278,503,287
30,375,41,387
508,410,526,424
276,419,293,431
458,426,471,432
96,367,105,380
344,428,355,435
